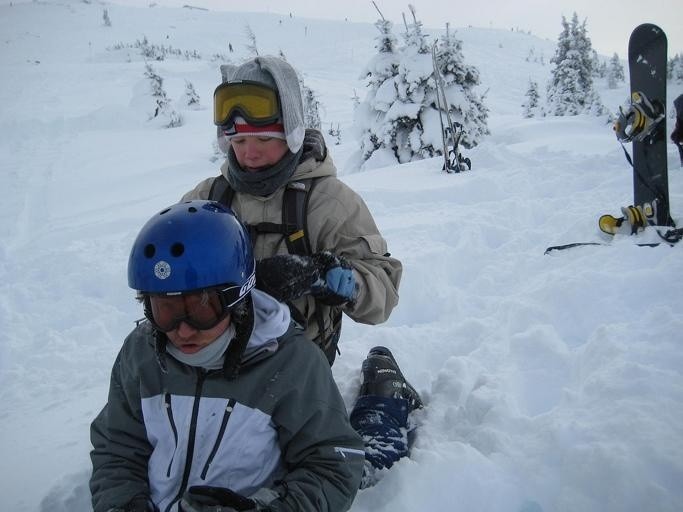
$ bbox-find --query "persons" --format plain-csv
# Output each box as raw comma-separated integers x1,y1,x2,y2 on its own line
90,197,366,512
181,50,421,489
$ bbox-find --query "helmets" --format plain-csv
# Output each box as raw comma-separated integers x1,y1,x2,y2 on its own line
128,199,257,293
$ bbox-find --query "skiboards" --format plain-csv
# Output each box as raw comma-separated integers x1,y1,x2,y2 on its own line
430,38,471,175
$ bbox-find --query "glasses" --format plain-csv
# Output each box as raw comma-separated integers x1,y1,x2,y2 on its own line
213,80,281,127
150,293,228,332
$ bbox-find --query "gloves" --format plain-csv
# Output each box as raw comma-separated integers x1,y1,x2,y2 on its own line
612,91,665,143
599,205,648,237
256,253,354,306
184,485,257,512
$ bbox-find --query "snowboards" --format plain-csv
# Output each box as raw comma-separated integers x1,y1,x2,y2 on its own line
627,23,670,227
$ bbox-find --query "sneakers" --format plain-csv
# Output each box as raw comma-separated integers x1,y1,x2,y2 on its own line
362,346,424,413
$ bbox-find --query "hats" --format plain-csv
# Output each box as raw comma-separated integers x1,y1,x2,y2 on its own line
217,56,306,154
